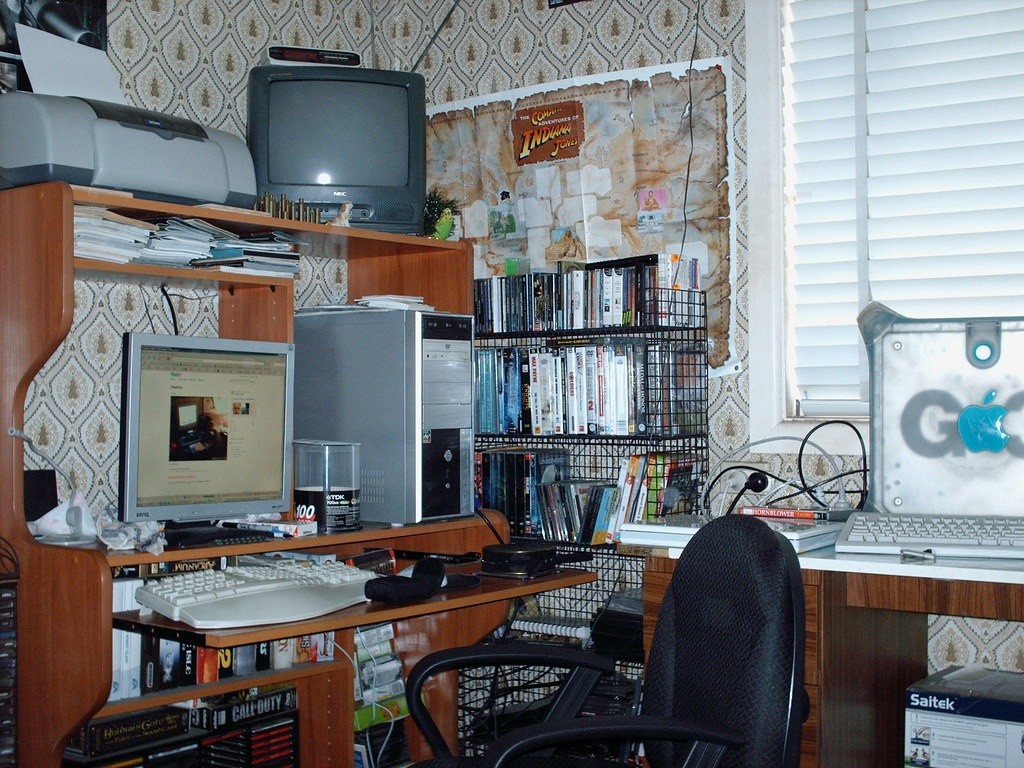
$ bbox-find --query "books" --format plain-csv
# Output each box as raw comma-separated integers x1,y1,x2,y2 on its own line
472,255,708,640
71,205,300,280
738,505,863,522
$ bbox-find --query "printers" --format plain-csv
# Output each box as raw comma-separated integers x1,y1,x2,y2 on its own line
0,61,256,209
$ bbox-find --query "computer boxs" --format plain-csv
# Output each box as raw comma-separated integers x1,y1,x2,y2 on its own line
291,310,474,525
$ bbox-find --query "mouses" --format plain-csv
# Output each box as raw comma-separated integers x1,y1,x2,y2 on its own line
399,565,448,588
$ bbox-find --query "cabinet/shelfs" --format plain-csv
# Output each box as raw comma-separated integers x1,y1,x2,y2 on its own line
0,180,598,768
456,286,712,766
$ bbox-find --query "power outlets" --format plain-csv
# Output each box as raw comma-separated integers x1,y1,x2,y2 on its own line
721,461,770,494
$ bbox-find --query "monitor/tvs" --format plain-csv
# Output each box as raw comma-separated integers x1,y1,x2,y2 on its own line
121,333,296,545
246,67,426,237
871,317,1024,519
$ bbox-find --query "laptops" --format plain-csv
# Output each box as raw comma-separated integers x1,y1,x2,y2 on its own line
621,513,845,553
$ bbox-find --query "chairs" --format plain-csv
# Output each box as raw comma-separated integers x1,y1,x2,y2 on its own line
404,514,811,768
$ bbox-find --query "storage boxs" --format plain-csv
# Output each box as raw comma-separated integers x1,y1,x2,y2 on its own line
903,664,1024,768
352,621,426,732
60,687,298,768
236,521,318,538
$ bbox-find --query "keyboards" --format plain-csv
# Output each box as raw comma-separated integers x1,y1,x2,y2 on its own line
136,559,385,628
835,511,1024,559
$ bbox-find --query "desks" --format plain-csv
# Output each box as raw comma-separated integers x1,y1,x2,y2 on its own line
616,543,1024,768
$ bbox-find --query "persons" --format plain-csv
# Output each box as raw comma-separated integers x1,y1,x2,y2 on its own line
232,402,241,415
